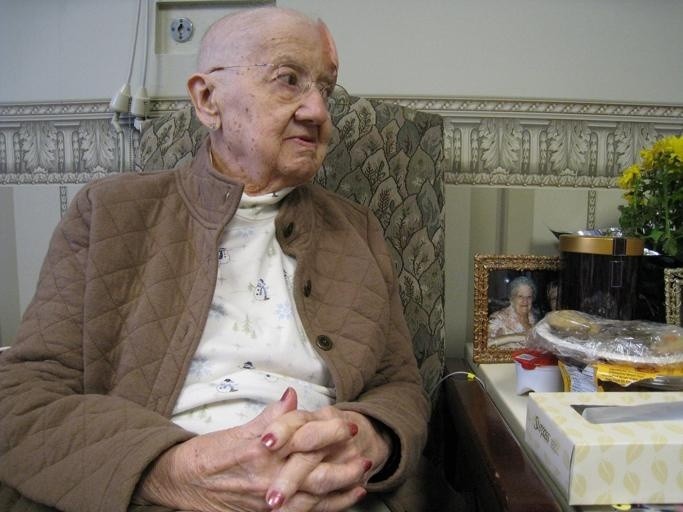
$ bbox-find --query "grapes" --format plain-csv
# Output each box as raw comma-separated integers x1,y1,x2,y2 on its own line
552,323,591,340
600,323,650,347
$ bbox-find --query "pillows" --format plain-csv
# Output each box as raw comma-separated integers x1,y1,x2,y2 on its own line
139,93,447,399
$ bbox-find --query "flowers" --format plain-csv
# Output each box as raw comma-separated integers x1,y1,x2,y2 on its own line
608,132,683,256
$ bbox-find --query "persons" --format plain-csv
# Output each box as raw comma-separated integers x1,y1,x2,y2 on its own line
1,6,432,512
491,277,539,348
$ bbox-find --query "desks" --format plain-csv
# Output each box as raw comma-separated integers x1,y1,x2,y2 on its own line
446,344,683,511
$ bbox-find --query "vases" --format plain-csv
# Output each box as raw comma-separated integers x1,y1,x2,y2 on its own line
633,256,683,324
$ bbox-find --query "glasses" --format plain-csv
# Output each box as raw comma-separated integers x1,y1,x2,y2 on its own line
207,63,351,117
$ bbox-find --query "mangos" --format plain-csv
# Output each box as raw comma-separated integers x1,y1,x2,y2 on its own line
650,334,683,356
549,310,598,336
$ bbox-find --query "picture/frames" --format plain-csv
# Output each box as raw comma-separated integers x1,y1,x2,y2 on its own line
472,253,563,363
